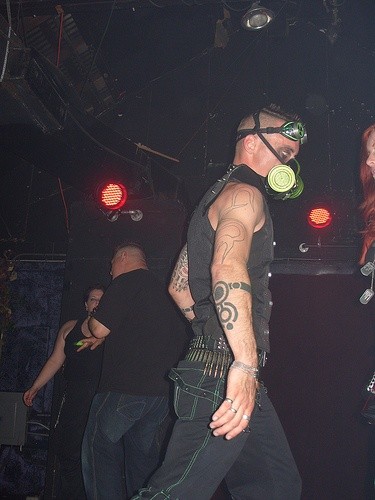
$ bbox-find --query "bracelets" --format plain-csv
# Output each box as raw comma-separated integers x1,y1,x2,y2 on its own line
231,360,260,380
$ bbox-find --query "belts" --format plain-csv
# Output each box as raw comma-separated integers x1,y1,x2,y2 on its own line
189,335,266,367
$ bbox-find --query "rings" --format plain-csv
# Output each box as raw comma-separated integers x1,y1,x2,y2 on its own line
229,407,237,414
242,414,251,421
225,398,233,403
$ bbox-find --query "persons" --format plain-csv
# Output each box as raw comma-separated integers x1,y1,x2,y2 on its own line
359,123,375,267
144,102,304,500
81,245,194,500
24,285,106,500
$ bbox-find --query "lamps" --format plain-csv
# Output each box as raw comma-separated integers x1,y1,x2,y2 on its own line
90,173,129,211
305,198,335,229
241,3,275,31
